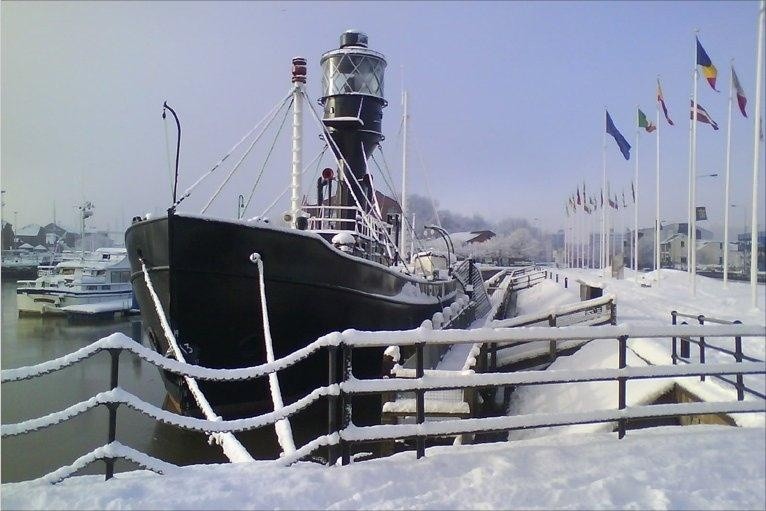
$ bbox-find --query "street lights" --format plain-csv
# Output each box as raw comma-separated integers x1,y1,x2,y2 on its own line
730,203,747,282
653,219,668,270
687,172,718,285
532,217,544,245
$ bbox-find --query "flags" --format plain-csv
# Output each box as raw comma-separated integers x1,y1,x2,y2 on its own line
565,184,636,215
697,38,721,94
639,110,657,134
606,111,632,161
733,67,749,119
658,87,675,127
691,100,719,131
